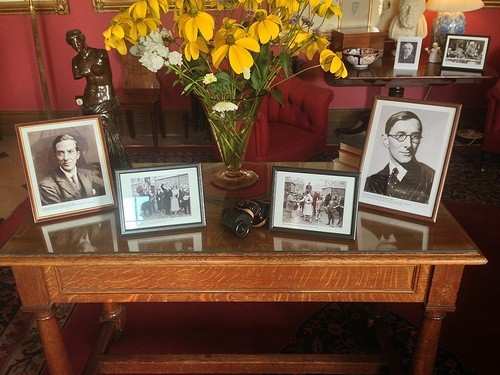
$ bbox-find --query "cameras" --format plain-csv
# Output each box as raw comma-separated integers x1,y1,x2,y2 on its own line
219,192,271,236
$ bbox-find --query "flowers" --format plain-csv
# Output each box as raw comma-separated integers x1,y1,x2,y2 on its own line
102,0,348,167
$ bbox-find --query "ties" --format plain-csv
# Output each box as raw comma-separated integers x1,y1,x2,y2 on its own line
71,175,81,196
387,167,400,197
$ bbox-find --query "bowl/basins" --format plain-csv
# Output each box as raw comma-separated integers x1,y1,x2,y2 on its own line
343,48,381,68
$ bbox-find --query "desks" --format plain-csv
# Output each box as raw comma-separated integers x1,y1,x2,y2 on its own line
325,56,500,136
1,160,489,375
115,88,165,146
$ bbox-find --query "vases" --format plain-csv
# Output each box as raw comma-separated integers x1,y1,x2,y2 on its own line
191,87,268,191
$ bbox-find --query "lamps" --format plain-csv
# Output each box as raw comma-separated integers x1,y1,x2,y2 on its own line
426,0,484,50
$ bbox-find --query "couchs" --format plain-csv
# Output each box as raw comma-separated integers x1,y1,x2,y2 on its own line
207,60,334,161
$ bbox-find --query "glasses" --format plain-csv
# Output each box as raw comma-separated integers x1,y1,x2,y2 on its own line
388,131,422,142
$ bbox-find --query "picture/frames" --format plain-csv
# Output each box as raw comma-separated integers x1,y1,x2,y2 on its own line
269,166,362,240
14,114,117,223
115,162,206,235
355,208,431,251
272,234,349,250
441,34,490,72
39,211,119,252
359,95,461,224
124,231,204,252
393,36,422,70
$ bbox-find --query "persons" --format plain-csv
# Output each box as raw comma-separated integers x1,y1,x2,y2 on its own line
390,0,427,41
38,134,104,205
363,111,435,204
66,29,127,171
137,182,190,218
398,42,415,63
285,184,345,228
449,41,481,64
